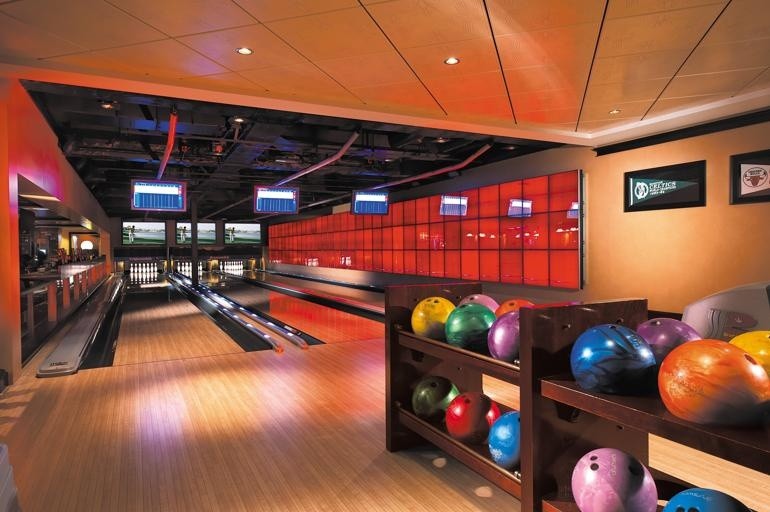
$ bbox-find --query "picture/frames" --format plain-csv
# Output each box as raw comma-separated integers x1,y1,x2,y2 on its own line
727,149,768,205
621,158,707,212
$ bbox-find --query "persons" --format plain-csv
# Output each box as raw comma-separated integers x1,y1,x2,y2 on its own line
229,227,234,244
127,225,135,244
179,225,186,243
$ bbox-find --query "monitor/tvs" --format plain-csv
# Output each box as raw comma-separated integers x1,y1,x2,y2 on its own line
251,184,299,218
439,194,469,219
349,188,389,216
565,200,580,221
506,197,533,219
129,178,189,215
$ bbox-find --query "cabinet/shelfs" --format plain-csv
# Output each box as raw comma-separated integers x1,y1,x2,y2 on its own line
382,281,769,511
